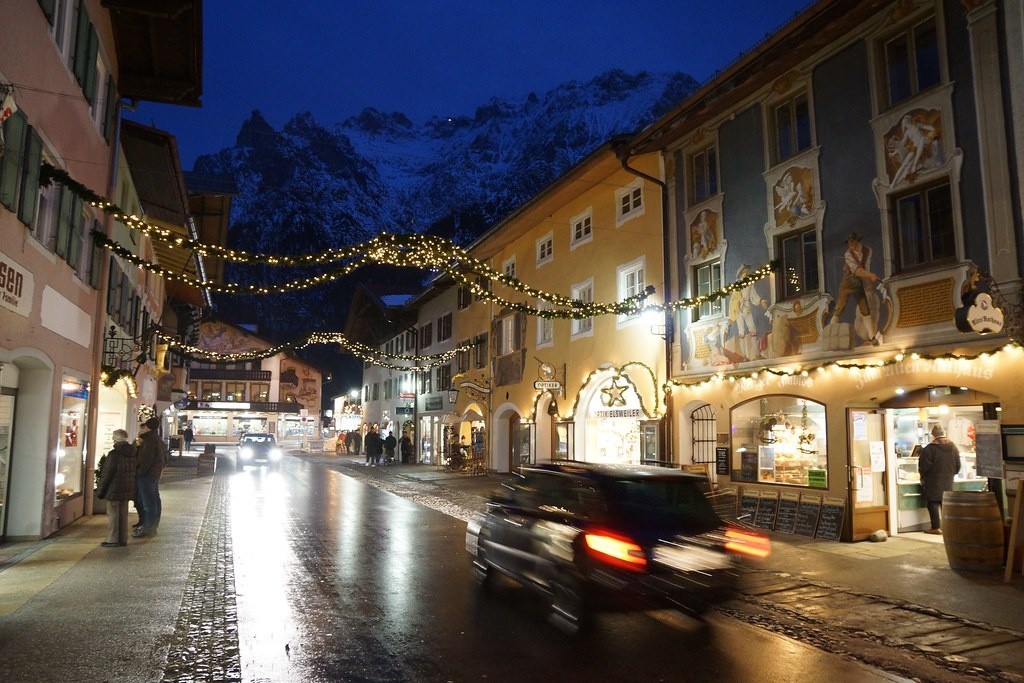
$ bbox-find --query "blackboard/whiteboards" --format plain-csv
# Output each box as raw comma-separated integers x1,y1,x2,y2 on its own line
740,452,759,483
169,437,182,450
975,434,1004,480
741,493,846,542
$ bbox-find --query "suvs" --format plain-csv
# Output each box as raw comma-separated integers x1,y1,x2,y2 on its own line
465,458,735,634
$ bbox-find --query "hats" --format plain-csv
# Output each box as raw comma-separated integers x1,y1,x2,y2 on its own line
931,425,943,436
145,418,160,430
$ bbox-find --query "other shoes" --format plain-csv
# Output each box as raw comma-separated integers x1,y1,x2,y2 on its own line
136,527,157,534
132,521,142,527
132,531,154,538
102,542,120,546
120,543,127,545
924,529,941,534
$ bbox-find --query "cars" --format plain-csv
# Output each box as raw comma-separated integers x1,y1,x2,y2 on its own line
235,433,283,470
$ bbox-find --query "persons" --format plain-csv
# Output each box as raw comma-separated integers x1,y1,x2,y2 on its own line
178,426,184,435
399,431,411,464
184,425,193,452
336,426,385,466
130,430,150,528
132,417,166,538
918,423,961,535
97,429,136,547
384,431,396,462
66,419,78,446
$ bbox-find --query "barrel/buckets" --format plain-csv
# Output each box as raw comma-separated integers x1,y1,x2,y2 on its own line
941,491,1005,570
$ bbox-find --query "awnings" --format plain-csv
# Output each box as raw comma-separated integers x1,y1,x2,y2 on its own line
107,0,205,110
117,117,214,311
